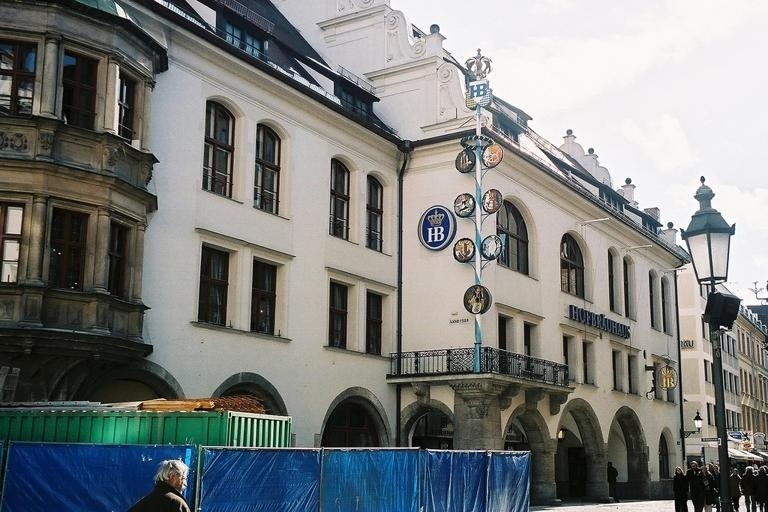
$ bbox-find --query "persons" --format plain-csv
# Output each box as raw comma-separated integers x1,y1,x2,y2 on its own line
125,457,192,512
607,460,618,500
671,459,767,512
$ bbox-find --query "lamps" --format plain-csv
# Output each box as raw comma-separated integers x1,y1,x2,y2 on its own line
680,410,704,439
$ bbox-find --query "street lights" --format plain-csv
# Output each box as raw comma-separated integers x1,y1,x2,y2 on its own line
677,174,738,511
